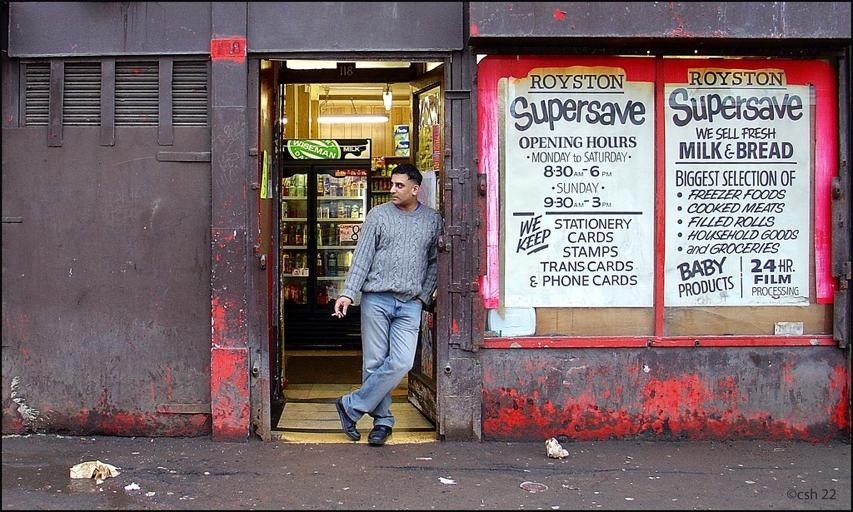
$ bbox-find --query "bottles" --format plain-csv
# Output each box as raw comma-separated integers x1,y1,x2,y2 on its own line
282,169,359,304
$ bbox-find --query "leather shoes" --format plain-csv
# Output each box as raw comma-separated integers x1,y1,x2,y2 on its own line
368,425,392,444
336,398,361,440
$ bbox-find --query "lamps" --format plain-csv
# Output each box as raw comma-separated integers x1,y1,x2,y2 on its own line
317,95,390,123
381,80,394,113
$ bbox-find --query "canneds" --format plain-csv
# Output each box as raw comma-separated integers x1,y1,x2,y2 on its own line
381,162,398,177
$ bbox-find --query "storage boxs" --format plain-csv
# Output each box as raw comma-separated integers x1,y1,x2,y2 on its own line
390,124,409,141
392,140,411,157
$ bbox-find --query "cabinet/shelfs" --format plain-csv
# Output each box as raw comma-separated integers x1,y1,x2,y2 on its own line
371,156,410,194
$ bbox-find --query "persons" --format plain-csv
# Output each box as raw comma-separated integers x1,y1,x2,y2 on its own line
332,163,440,448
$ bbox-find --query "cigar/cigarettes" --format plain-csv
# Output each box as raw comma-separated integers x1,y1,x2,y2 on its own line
329,311,340,317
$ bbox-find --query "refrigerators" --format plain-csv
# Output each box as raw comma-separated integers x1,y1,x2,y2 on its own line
279,138,371,350
